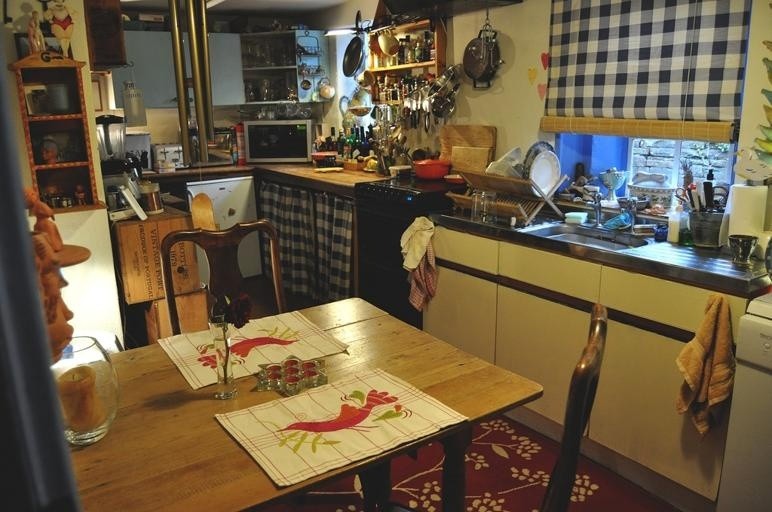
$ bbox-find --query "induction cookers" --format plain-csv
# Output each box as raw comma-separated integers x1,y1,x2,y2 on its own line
354,175,467,210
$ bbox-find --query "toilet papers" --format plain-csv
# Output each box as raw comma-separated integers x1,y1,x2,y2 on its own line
717,185,768,249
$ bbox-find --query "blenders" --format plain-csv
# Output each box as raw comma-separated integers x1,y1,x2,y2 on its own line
95,113,142,222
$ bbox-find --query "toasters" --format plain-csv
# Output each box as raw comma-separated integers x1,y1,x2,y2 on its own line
150,142,185,170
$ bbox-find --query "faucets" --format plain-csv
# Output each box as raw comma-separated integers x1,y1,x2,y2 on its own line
620,197,637,235
586,192,604,228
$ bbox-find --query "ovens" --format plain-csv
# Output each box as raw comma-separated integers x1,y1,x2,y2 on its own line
357,205,424,331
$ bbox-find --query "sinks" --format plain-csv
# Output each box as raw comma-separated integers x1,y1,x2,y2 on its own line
518,222,648,251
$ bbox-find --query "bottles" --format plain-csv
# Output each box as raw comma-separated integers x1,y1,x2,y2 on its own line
319,125,373,160
676,204,688,229
40,182,87,209
374,30,436,67
374,75,431,101
243,77,280,102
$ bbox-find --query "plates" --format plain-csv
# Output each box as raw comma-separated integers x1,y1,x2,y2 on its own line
444,174,465,184
529,151,561,198
523,139,555,192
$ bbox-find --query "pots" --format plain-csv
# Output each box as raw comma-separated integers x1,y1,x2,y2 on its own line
397,159,451,181
463,29,504,89
343,8,364,77
425,63,463,111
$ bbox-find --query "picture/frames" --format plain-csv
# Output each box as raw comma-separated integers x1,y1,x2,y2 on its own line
13,32,73,61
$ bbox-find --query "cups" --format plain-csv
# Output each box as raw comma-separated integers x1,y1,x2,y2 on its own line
727,234,759,267
139,183,165,215
470,192,498,225
208,321,239,399
583,185,600,204
667,213,680,243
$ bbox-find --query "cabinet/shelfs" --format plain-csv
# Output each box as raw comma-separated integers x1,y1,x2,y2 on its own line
420,219,498,366
112,30,245,109
587,248,749,512
239,31,328,104
12,52,107,213
497,239,602,452
84,0,126,70
368,18,446,107
185,176,262,288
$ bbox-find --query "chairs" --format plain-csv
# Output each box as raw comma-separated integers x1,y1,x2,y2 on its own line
158,218,286,337
540,304,610,511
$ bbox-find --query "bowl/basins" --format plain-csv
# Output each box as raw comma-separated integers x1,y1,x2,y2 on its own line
627,183,675,209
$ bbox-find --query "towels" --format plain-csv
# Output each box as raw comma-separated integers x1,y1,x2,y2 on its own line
157,310,351,392
407,242,437,311
399,216,434,269
674,293,736,436
213,369,471,488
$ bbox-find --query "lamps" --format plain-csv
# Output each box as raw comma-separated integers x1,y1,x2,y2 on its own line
322,28,371,36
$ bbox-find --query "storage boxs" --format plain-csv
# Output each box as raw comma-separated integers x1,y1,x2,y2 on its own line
112,207,203,306
146,284,208,343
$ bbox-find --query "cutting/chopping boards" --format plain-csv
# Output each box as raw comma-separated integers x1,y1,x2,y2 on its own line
439,125,497,173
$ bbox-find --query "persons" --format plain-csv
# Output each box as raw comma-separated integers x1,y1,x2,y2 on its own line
44,0,78,59
27,11,40,54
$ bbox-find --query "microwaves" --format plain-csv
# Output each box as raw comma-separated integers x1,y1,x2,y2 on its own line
180,126,233,169
